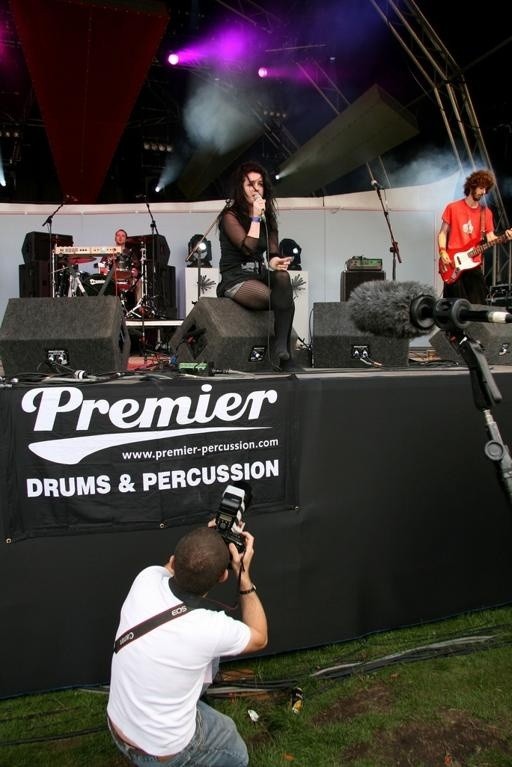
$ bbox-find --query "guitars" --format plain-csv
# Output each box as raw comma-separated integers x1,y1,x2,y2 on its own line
439,228,508,286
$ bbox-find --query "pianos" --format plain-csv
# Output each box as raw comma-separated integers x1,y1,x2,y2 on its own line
55,246,123,255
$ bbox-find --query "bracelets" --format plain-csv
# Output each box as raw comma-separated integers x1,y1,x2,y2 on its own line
439,246,446,254
252,216,262,222
239,583,257,594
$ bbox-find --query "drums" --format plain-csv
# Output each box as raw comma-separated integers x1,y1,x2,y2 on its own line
113,255,132,279
115,278,130,291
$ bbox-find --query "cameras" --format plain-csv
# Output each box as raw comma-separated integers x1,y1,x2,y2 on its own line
210,481,252,560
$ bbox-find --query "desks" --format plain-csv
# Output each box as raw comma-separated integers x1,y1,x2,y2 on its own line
176,267,309,349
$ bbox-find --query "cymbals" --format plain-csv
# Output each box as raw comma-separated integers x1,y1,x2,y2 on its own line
64,257,96,263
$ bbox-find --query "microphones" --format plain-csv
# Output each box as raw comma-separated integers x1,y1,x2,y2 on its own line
253,193,269,222
371,180,384,191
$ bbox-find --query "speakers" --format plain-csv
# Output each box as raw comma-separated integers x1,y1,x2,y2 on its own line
123,236,169,265
169,296,298,373
340,270,385,302
0,297,132,379
18,262,57,296
429,304,512,366
311,302,409,368
20,231,73,259
151,267,179,318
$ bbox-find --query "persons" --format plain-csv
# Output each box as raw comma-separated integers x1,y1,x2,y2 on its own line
217,159,297,368
436,169,512,341
106,513,269,766
98,228,149,314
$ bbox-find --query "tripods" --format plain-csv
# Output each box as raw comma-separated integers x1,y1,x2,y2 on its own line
123,261,161,320
